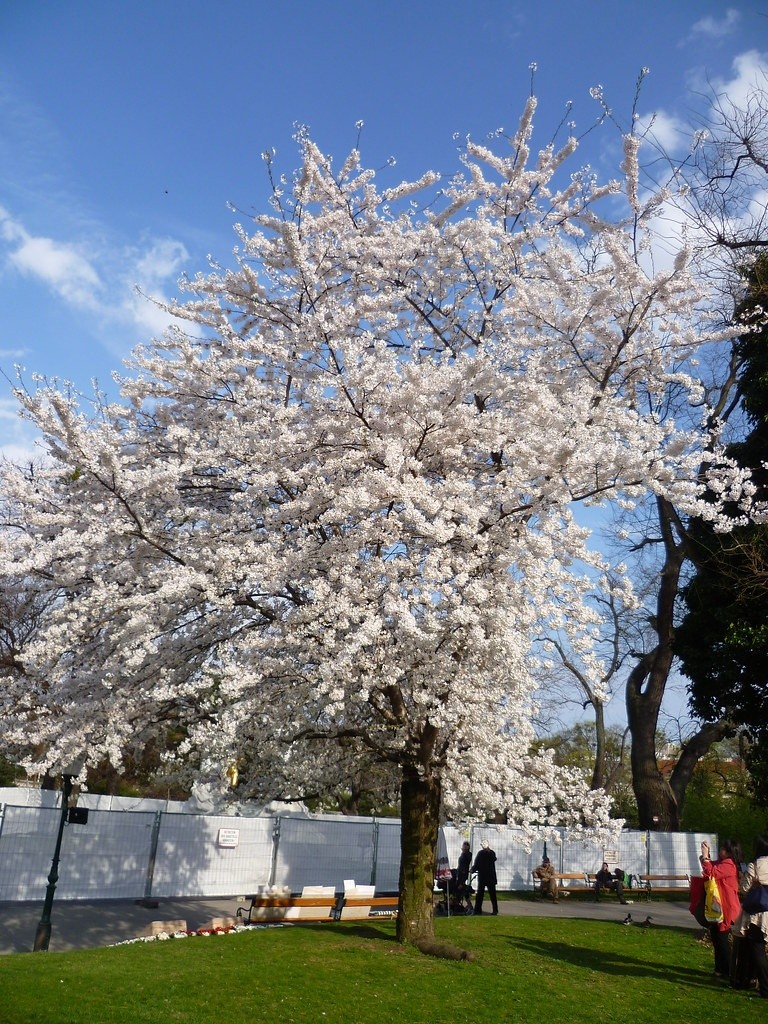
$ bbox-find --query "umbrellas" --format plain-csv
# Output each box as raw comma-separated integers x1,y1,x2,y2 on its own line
434,822,453,917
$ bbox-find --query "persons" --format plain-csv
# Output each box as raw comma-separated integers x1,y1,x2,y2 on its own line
697,834,768,998
470,839,500,915
456,842,474,912
595,862,630,905
532,856,558,905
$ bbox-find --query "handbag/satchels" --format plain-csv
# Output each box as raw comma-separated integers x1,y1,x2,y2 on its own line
742,861,768,915
702,876,724,924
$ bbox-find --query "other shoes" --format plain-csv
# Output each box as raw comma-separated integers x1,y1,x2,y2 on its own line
553,898,558,904
547,891,553,897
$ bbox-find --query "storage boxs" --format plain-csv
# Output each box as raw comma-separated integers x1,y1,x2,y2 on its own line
136,919,187,936
196,916,244,931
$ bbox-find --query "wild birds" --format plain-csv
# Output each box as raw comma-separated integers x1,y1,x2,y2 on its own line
640,916,653,928
622,913,634,925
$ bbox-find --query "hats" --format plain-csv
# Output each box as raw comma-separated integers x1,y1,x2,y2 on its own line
542,858,550,863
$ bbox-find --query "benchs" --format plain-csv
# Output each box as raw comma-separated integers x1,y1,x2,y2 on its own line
330,897,399,920
533,872,692,902
235,897,340,922
